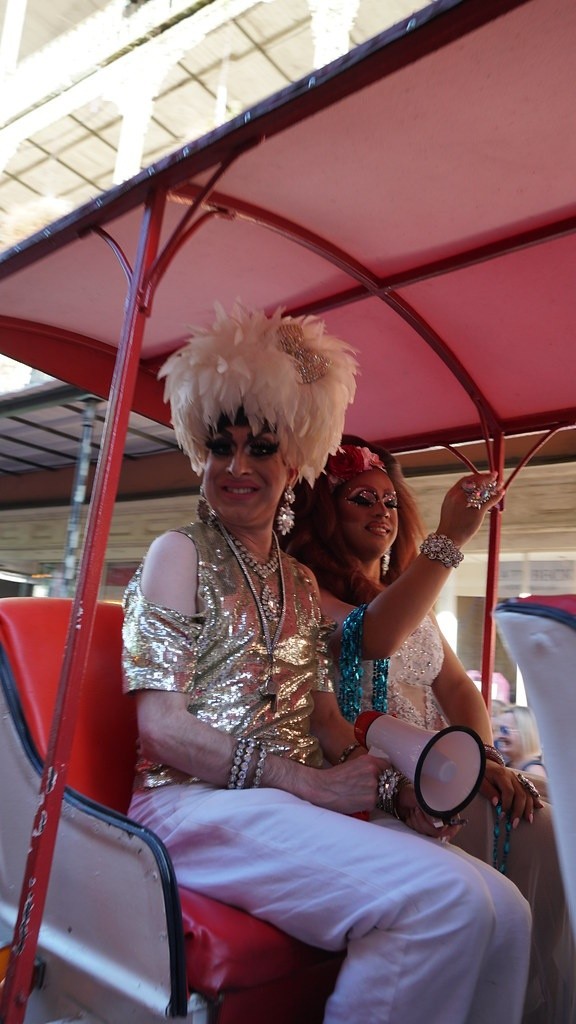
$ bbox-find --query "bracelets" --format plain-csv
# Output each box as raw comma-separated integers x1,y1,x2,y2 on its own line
420,533,464,568
338,742,361,762
227,737,268,790
377,767,411,821
483,743,505,767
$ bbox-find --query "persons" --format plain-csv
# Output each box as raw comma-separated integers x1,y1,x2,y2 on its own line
280,434,575,1024
489,699,546,779
120,307,534,1024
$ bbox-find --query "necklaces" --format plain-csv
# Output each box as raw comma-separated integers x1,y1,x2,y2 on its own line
491,801,512,872
219,522,286,712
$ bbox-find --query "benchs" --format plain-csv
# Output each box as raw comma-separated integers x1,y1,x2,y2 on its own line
0,597,345,1024
491,593,576,938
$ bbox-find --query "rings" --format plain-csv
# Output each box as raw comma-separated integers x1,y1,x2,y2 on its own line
466,480,498,510
517,774,541,798
462,481,476,492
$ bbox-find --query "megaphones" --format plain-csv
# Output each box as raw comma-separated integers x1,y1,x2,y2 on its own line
347,710,487,822
